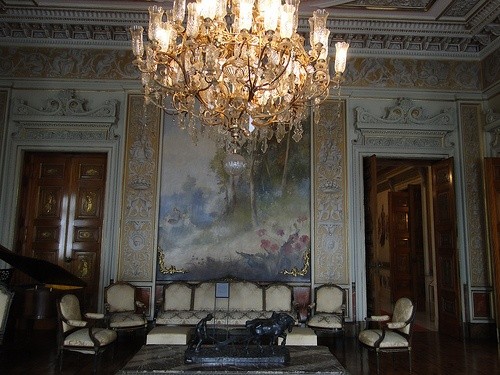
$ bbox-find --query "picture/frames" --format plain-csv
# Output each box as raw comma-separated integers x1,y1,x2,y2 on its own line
154,94,314,288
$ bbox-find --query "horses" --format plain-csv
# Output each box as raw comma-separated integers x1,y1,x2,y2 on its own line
245,311,297,355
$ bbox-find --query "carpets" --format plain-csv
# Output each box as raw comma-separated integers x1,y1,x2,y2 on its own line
115,344,346,375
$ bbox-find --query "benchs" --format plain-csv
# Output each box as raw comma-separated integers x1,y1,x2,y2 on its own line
153,274,302,328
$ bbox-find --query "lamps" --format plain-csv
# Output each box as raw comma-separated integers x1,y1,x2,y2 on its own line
128,0,350,153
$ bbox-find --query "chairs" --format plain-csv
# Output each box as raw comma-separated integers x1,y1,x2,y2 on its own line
358,296,417,375
55,291,119,375
0,285,15,341
304,282,347,351
103,282,149,345
